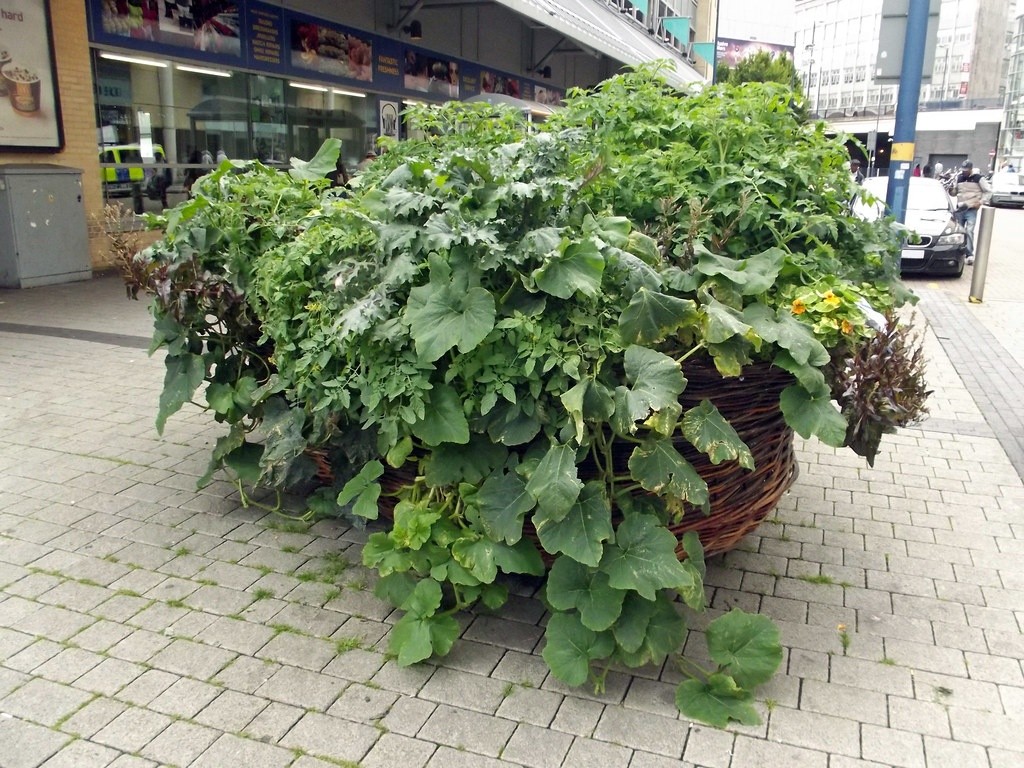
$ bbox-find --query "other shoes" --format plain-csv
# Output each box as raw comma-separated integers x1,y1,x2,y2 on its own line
966,256,974,265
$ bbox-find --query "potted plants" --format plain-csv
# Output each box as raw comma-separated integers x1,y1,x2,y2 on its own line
91,60,928,728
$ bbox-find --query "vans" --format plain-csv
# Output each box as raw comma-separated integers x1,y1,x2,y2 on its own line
99,142,166,191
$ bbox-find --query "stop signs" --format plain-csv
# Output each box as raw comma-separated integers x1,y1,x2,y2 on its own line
988,150,996,158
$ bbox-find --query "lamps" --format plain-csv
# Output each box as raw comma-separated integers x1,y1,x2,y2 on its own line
536,66,552,78
403,20,422,40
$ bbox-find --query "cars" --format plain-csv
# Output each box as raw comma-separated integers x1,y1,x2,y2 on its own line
984,171,1024,209
845,175,973,279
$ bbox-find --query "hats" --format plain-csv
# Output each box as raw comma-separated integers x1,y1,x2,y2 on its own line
852,159,860,164
959,160,972,171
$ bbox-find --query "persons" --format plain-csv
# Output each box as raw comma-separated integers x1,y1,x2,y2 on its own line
1007,164,1015,172
948,160,993,267
843,159,864,187
913,160,944,180
